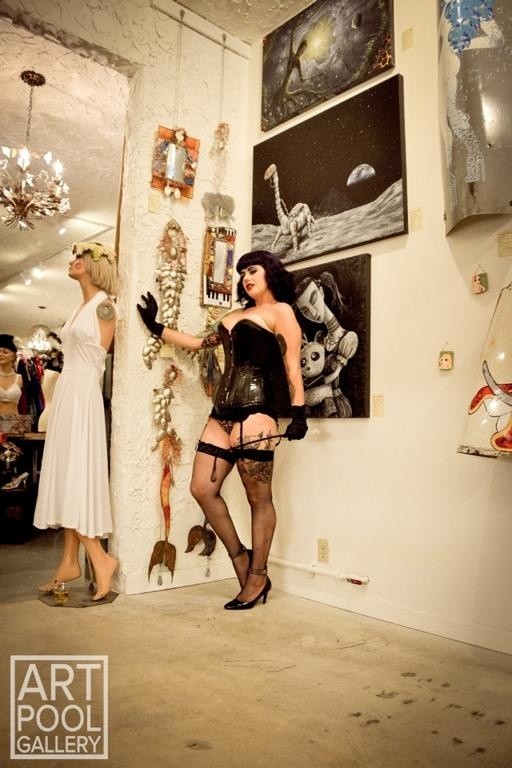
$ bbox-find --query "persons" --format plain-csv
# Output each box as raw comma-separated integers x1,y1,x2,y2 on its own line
137,250,308,611
289,271,359,417
0,334,23,414
32,241,120,601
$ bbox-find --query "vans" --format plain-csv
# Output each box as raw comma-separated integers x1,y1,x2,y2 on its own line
222,549,272,611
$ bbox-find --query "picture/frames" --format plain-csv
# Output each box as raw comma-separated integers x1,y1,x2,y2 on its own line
3,430,47,483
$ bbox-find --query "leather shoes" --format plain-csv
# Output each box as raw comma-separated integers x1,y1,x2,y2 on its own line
282,404,309,441
135,289,166,339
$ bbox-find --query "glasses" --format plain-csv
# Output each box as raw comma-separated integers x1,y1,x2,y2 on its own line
24,301,55,356
0,69,74,231
